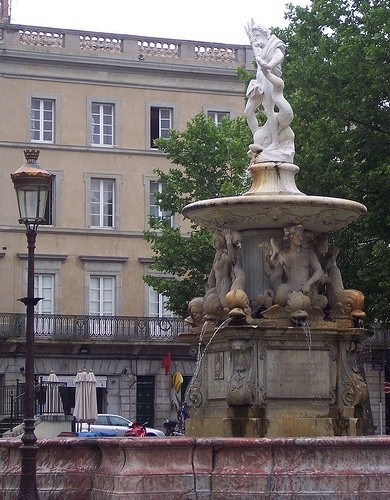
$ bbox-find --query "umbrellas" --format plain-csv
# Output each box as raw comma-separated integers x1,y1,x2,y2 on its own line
73,369,98,419
44,369,65,413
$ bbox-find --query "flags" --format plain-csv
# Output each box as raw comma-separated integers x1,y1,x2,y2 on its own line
164,351,172,375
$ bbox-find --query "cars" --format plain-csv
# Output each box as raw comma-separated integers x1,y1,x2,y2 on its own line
75,413,165,437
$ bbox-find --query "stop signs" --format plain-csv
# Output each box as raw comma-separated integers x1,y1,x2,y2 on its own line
383,382,390,393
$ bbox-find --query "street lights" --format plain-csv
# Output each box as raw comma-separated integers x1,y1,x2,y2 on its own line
8,150,51,500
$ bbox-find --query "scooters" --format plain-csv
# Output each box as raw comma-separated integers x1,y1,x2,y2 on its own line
125,421,148,437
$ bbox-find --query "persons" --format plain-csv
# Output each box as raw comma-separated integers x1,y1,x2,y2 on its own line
269,224,328,312
243,18,286,153
207,227,237,313
